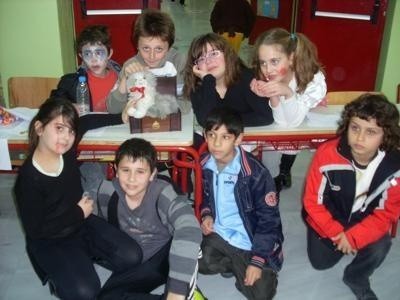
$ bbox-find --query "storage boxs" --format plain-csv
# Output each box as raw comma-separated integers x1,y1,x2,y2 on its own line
126,75,182,134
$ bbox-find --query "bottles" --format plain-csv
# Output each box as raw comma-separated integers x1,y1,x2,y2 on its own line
75,76,90,114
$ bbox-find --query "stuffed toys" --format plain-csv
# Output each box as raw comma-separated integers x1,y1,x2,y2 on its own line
126,70,178,120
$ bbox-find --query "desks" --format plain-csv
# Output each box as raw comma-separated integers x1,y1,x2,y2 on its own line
196,90,400,220
0,96,196,196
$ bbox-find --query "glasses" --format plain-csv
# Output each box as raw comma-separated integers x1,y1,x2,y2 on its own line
193,50,223,66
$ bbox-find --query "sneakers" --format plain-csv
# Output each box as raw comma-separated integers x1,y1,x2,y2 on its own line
341,274,376,300
273,174,292,191
189,285,206,300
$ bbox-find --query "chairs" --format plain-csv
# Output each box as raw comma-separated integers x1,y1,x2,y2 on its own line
7,77,61,107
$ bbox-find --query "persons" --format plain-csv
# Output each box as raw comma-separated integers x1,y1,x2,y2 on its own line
11,95,144,299
105,9,192,115
249,28,327,193
41,24,121,112
301,93,400,300
199,110,284,300
84,138,203,300
181,33,274,127
